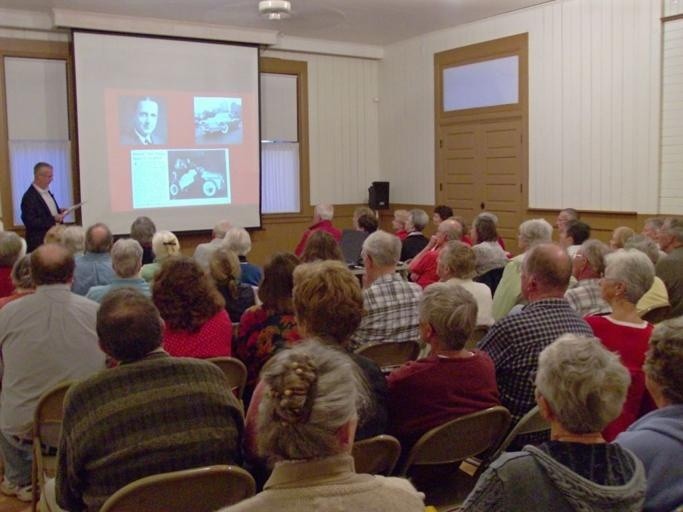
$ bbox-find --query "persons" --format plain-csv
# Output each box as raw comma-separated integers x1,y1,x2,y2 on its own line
21,161,70,254
2,203,683,512
177,157,194,180
129,98,159,144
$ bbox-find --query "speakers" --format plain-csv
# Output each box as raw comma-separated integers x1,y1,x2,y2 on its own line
368,181,390,211
